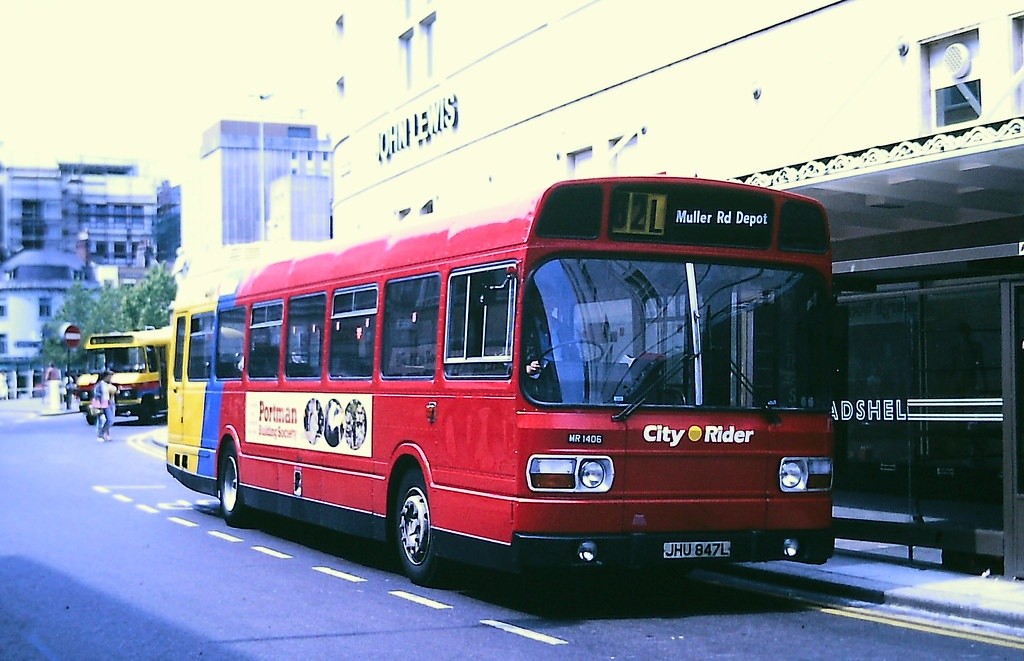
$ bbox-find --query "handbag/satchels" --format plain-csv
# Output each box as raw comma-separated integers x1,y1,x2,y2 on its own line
92,397,108,409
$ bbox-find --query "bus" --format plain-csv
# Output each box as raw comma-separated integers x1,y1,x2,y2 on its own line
76,324,170,423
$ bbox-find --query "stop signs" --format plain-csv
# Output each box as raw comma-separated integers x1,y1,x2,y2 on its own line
65,325,81,346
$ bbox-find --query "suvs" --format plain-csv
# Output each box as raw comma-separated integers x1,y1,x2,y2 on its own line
161,170,839,603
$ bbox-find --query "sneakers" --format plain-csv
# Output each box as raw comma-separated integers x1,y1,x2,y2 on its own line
98,433,112,443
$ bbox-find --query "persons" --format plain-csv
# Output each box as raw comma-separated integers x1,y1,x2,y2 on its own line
42,362,78,405
93,372,113,443
97,372,116,441
